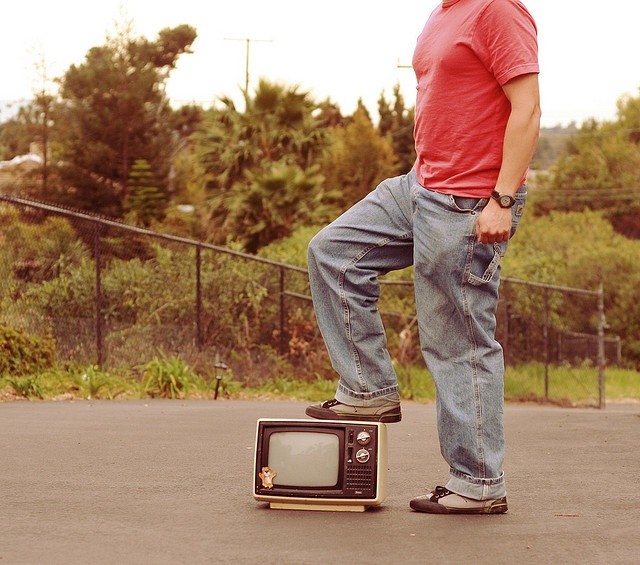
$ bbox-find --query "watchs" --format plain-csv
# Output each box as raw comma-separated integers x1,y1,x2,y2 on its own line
489,190,517,209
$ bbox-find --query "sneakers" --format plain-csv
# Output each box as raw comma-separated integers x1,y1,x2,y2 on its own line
409,485,508,514
305,398,401,422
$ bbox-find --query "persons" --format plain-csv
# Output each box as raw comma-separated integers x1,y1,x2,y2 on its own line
304,1,545,516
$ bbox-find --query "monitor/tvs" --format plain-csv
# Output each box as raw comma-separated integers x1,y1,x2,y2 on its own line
252,417,388,512
